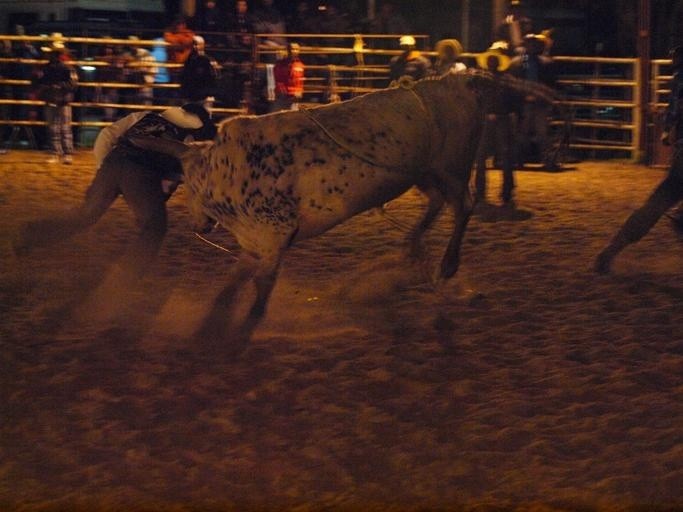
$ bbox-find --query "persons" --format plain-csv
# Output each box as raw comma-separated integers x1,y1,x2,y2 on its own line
499,1,565,171
661,46,682,222
390,35,466,85
594,157,682,273
473,51,515,205
2,1,400,165
13,102,219,328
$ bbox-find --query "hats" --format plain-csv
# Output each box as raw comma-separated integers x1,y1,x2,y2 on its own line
477,40,512,72
434,39,463,61
400,35,417,46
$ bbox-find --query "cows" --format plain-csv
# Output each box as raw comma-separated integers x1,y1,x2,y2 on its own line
129,68,572,363
